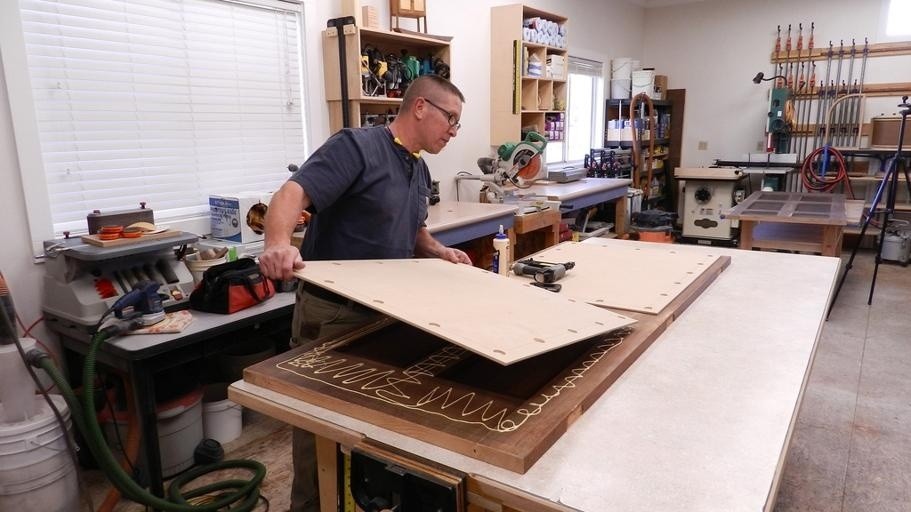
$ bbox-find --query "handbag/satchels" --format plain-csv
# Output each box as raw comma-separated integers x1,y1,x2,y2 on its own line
188,256,277,315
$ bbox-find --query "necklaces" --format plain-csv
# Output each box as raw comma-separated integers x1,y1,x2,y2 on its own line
386,125,396,141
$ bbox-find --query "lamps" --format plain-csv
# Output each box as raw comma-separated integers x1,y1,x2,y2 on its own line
752,72,789,89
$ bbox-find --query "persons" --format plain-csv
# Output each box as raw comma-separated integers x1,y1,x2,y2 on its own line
255,75,475,510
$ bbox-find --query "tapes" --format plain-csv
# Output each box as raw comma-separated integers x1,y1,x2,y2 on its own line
99,225,141,240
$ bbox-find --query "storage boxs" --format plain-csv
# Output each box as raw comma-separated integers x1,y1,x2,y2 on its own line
207,191,276,244
575,224,615,240
547,166,588,181
606,129,622,142
621,127,639,140
190,238,266,265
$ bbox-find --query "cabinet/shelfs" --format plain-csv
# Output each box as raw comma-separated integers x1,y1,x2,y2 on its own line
491,3,570,146
825,145,911,251
606,100,671,211
319,28,452,141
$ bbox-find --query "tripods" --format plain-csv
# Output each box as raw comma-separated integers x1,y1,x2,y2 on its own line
826,95,911,320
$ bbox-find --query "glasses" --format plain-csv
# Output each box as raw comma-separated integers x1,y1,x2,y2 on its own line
424,97,462,132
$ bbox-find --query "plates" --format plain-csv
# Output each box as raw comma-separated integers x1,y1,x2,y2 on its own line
82,230,183,249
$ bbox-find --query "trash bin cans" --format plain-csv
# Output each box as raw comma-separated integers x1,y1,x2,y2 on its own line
628,210,677,242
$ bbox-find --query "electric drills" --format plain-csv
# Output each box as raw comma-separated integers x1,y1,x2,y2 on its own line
514,257,577,285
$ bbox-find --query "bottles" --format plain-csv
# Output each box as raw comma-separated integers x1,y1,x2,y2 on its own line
405,56,421,81
492,224,512,278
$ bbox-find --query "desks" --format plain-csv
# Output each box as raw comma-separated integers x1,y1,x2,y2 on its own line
49,288,300,498
506,176,635,242
720,189,849,257
515,202,562,252
423,200,519,263
226,238,846,512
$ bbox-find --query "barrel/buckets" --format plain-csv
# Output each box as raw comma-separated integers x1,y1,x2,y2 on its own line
202,382,243,445
610,57,656,100
1,392,81,512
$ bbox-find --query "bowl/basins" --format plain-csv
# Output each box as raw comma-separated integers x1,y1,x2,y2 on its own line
94,378,206,479
227,340,273,377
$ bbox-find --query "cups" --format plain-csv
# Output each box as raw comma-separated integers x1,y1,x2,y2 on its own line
184,254,228,286
